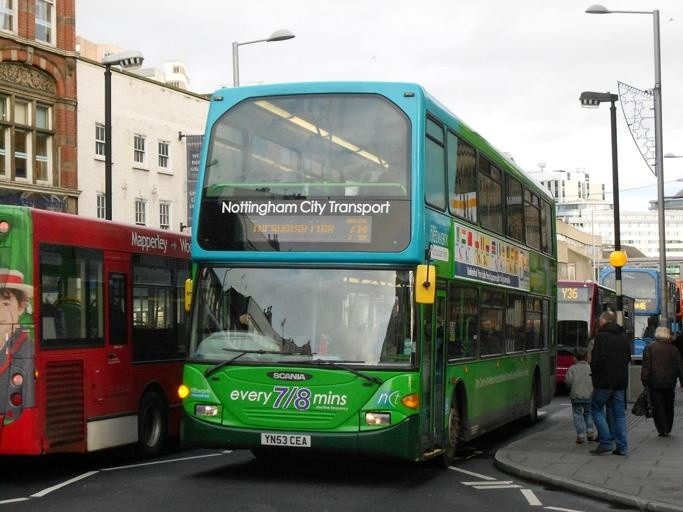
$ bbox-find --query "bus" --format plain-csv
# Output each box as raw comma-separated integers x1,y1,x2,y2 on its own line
0,202,199,466
554,279,635,388
597,268,675,359
177,72,557,487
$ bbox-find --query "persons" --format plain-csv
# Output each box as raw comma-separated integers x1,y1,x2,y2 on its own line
584,325,600,363
644,324,683,360
383,310,541,368
640,326,682,437
564,346,597,444
0,268,33,424
589,311,631,456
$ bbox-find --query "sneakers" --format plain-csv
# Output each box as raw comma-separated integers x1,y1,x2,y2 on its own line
613,448,628,455
576,431,594,443
590,445,611,453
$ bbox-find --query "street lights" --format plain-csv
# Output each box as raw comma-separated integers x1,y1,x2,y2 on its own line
586,5,670,333
100,51,143,221
579,90,630,408
230,29,296,96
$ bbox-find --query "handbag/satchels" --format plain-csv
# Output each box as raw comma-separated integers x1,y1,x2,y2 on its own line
632,387,654,418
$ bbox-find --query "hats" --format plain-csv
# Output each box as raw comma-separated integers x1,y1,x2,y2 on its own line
1,268,35,298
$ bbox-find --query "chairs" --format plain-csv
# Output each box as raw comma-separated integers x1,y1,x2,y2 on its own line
50,297,125,342
